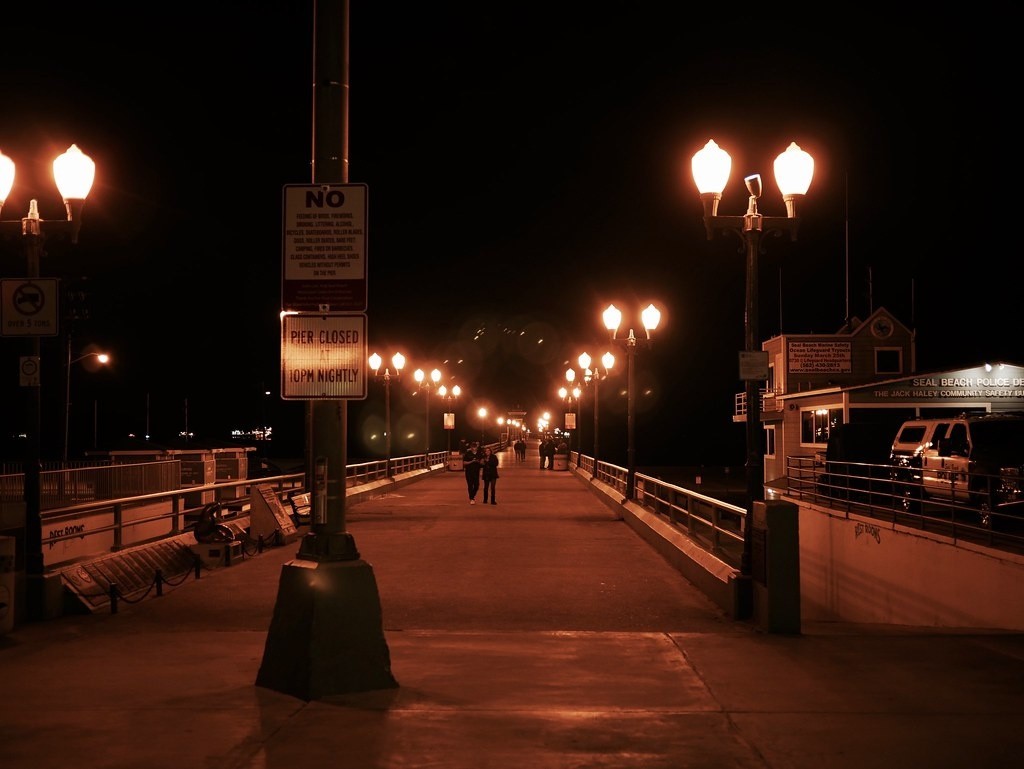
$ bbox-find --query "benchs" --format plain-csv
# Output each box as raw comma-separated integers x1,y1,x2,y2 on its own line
288,487,314,526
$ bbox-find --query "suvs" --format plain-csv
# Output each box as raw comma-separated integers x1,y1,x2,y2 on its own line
889,410,1024,530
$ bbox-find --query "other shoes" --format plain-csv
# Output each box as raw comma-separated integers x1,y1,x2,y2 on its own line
540,467,546,469
470,499,475,504
483,499,487,503
548,468,553,470
491,501,497,505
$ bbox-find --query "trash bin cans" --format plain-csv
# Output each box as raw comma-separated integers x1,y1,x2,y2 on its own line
553,454,568,470
752,498,802,633
448,455,464,471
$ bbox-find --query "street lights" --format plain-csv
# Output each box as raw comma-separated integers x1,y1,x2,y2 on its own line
369,351,406,480
414,368,442,469
63,338,110,496
578,351,615,478
558,386,582,462
690,137,817,622
601,302,661,501
438,384,461,464
478,408,486,448
497,416,504,452
0,141,97,628
566,368,593,467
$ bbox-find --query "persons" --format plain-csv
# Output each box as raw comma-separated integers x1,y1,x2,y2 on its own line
463,443,483,504
514,440,526,460
546,439,555,470
539,440,547,469
480,446,499,505
459,438,484,467
